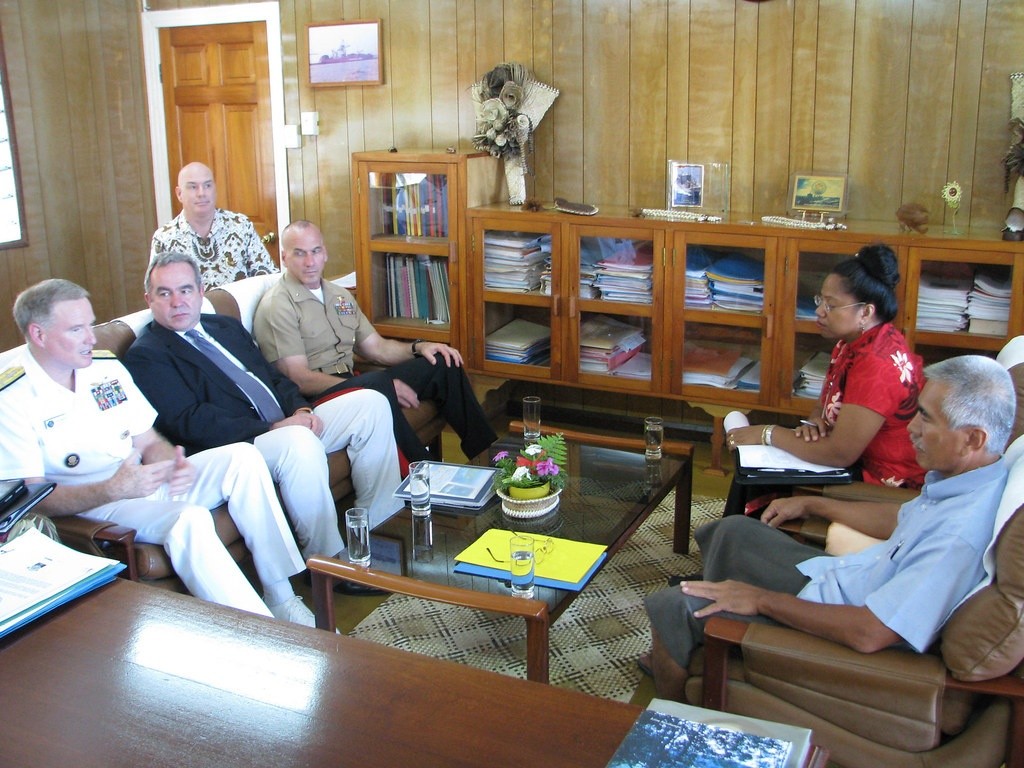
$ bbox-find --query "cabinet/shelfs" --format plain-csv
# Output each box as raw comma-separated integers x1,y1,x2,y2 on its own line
470,200,780,416
346,150,505,385
781,210,1024,434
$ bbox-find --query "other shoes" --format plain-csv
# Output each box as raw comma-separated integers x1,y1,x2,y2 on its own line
667,573,705,586
638,651,658,680
337,580,391,596
262,597,342,634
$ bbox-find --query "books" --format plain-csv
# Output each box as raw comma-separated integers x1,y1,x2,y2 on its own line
606,697,812,768
392,460,502,510
483,231,832,399
368,171,451,323
915,273,1012,336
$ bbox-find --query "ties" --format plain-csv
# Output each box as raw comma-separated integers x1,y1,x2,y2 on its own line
185,329,284,426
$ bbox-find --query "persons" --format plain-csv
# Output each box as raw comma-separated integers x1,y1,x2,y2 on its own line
252,219,499,465
151,162,280,293
638,355,1016,705
0,277,315,628
669,243,927,452
121,252,405,597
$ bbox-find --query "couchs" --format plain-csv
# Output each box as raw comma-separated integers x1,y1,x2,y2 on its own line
0,270,453,595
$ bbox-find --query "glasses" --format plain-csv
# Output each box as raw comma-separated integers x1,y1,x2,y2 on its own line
812,293,867,314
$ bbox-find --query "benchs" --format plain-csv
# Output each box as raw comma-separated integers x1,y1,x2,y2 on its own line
684,332,1024,768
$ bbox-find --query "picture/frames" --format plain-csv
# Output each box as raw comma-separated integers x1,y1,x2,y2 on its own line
784,171,854,216
665,161,706,211
303,20,384,92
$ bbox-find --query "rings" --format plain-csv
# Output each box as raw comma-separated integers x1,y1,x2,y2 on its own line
731,434,733,438
730,440,734,445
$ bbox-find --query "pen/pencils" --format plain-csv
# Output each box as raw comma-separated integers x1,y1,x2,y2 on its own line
800,420,817,426
890,540,904,559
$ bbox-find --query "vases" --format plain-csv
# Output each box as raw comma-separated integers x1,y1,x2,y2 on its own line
494,481,560,518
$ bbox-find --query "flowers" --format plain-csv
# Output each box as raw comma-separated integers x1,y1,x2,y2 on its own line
465,60,559,201
939,179,971,240
491,432,571,488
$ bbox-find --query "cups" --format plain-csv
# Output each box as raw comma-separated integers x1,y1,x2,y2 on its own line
512,535,534,599
522,396,544,441
410,515,432,563
345,508,371,561
410,462,430,517
644,416,664,459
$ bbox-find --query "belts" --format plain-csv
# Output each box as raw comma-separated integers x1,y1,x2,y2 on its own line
312,362,357,377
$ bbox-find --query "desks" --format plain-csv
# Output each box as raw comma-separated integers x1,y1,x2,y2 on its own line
306,419,696,684
0,578,832,768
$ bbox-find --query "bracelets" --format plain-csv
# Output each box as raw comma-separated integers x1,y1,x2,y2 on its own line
761,424,778,445
